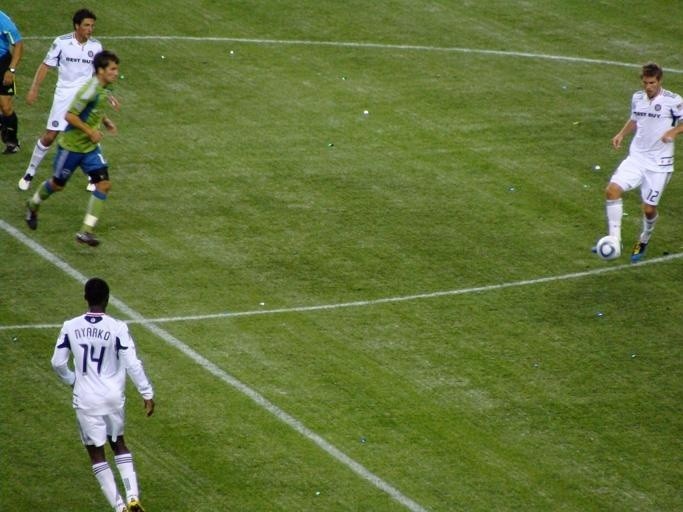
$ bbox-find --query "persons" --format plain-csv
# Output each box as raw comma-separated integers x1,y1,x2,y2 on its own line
589,61,682,262
23,50,119,248
16,8,120,193
0,8,23,155
50,277,155,512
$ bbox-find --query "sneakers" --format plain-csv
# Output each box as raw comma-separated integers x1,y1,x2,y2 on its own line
86,176,96,192
128,498,146,512
630,239,647,263
7,143,20,153
18,168,36,191
76,230,101,247
24,199,39,231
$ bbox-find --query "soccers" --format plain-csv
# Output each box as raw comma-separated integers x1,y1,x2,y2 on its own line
595,236,624,260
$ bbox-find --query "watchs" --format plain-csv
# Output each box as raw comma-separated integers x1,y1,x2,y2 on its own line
5,67,16,74
